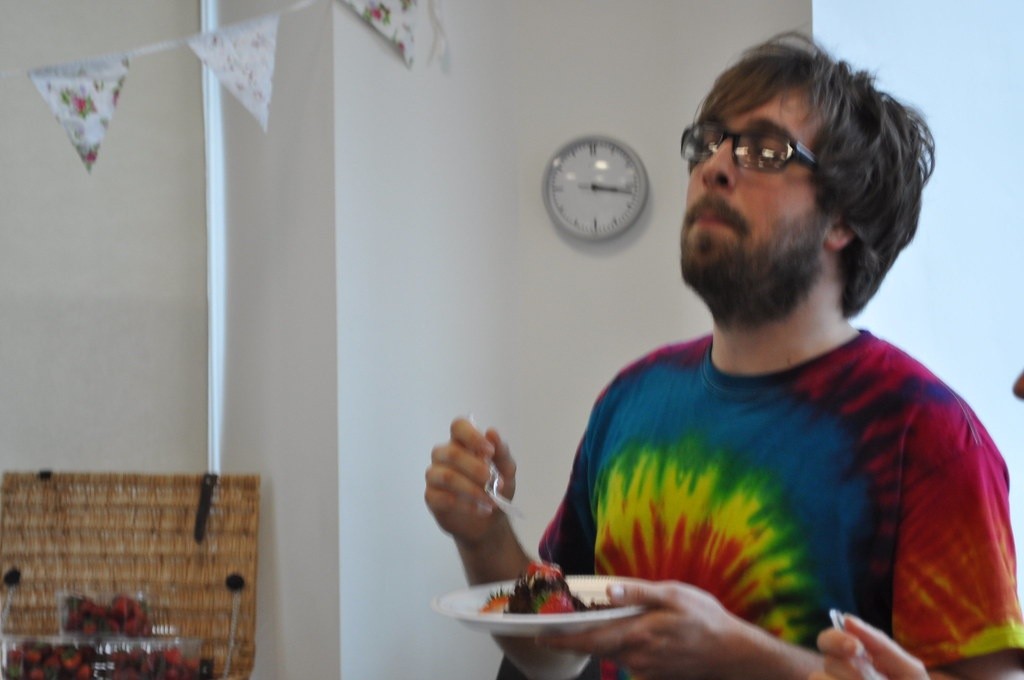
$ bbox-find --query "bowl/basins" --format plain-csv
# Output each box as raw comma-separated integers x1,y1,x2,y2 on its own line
0,634,203,680
57,591,153,638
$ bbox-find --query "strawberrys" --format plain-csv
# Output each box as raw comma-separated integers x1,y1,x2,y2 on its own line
6,596,198,680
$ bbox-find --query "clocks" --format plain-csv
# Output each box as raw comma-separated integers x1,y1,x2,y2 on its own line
541,134,647,242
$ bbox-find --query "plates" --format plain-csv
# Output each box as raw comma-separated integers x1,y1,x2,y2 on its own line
430,573,653,637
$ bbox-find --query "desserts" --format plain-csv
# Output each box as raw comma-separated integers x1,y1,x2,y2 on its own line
481,563,612,616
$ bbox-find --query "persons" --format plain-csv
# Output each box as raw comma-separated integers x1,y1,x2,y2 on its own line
420,35,1022,679
817,372,1023,680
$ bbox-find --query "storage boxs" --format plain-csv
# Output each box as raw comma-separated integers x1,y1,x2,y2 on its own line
0,634,95,679
55,590,152,639
96,638,201,680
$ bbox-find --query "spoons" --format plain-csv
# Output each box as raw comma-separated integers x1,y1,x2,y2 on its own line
469,415,525,521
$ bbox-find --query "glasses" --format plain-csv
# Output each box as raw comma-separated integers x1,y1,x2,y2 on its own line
681,122,817,173
